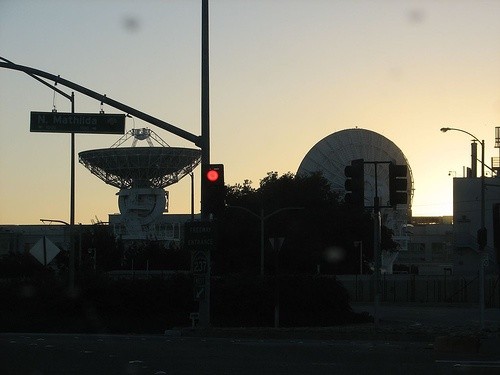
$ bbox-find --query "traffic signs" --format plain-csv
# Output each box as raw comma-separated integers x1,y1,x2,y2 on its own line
30,110,125,134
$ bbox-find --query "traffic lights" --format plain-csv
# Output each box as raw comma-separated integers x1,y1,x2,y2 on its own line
389,164,407,204
345,159,364,199
206,164,224,212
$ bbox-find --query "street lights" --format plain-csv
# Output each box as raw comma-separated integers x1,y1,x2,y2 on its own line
440,127,485,329
